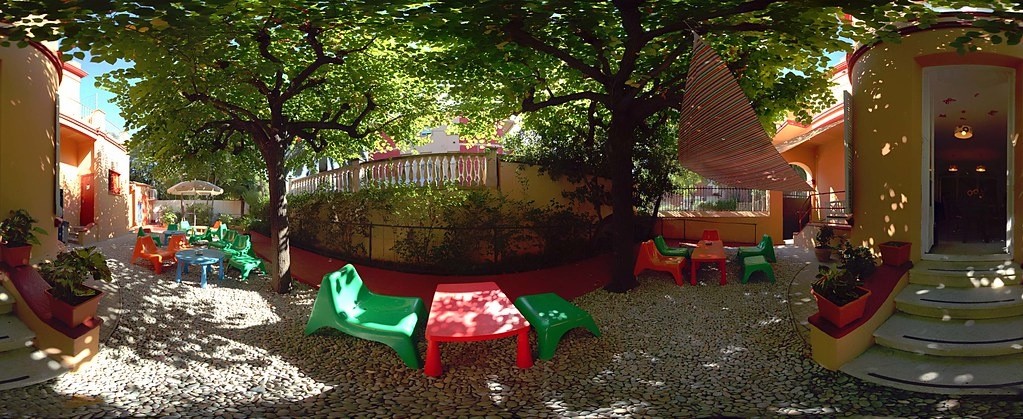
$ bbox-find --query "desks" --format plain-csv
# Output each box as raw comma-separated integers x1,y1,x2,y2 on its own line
190,226,206,234
422,279,534,377
690,240,726,286
162,230,183,245
174,248,226,288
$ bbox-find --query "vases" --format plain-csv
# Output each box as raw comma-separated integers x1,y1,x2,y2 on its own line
877,241,911,267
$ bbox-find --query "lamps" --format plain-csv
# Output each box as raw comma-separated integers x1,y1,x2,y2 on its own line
948,165,958,172
952,124,973,139
975,165,985,172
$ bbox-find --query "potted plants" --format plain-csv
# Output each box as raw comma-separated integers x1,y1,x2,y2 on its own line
833,235,877,277
35,245,112,329
810,263,873,327
813,225,834,263
0,209,48,268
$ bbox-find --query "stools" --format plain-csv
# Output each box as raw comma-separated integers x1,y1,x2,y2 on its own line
739,254,775,284
515,292,601,360
226,254,266,279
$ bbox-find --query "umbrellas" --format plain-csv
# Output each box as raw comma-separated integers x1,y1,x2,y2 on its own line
167,178,224,226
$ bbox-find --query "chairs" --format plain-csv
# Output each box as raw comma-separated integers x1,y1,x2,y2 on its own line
652,235,689,258
303,264,428,369
131,219,265,287
700,229,720,241
735,233,776,263
633,239,689,285
